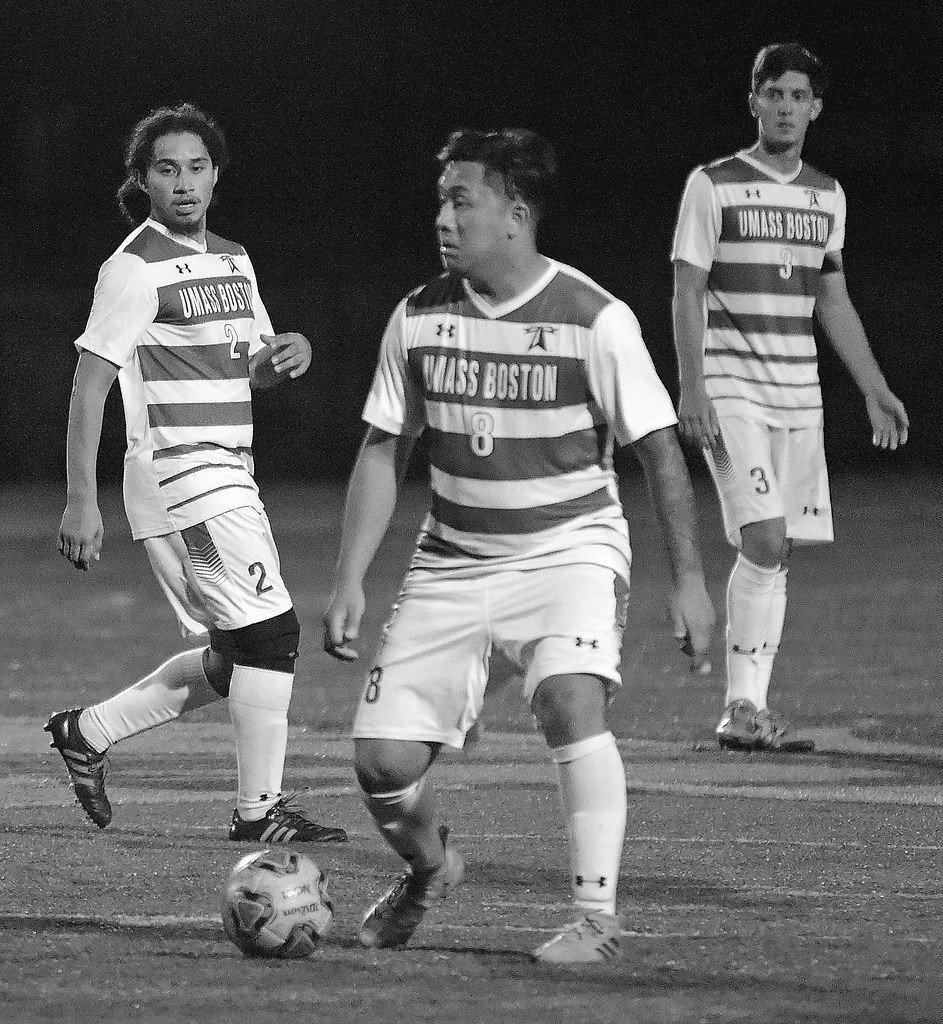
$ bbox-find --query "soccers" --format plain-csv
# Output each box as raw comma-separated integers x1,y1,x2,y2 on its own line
219,846,336,961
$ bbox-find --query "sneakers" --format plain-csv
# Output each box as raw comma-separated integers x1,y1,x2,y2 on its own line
529,908,622,963
228,786,347,844
715,698,762,751
43,707,112,830
359,824,463,949
758,709,814,752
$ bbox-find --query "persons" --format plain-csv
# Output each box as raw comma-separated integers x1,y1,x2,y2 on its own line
670,43,909,752
322,129,717,964
42,104,352,841
